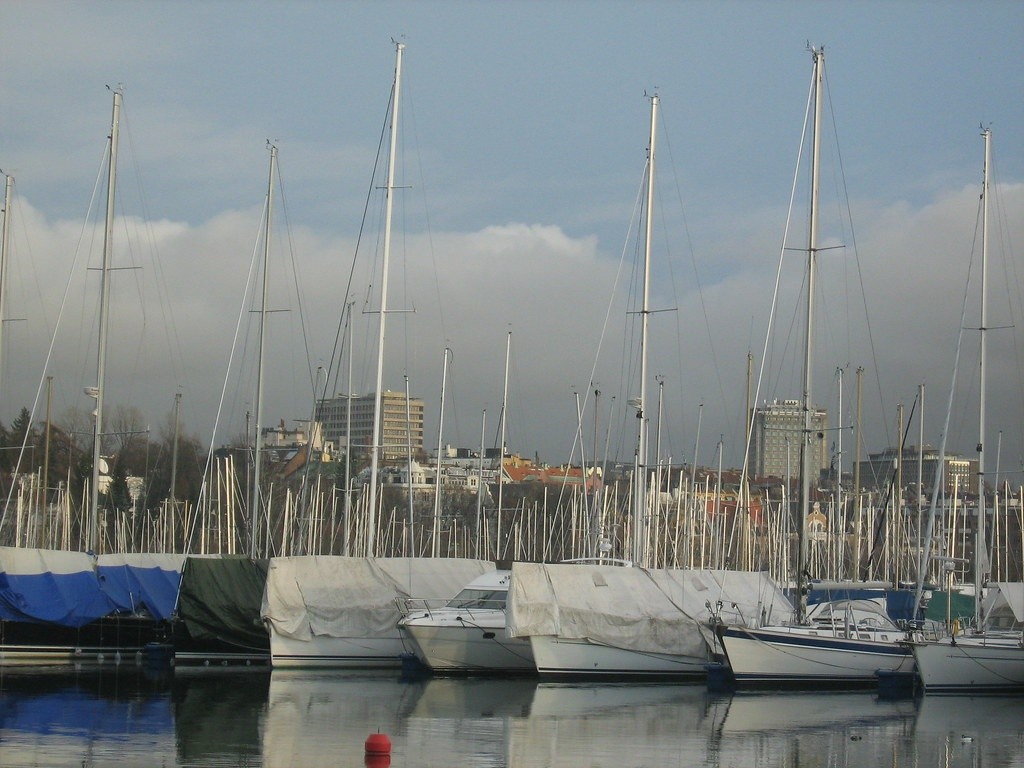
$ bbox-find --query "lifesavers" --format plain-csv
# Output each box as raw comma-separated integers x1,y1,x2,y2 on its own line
952,619,960,634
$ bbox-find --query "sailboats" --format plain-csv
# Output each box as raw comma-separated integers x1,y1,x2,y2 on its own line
0,36,1024,694
